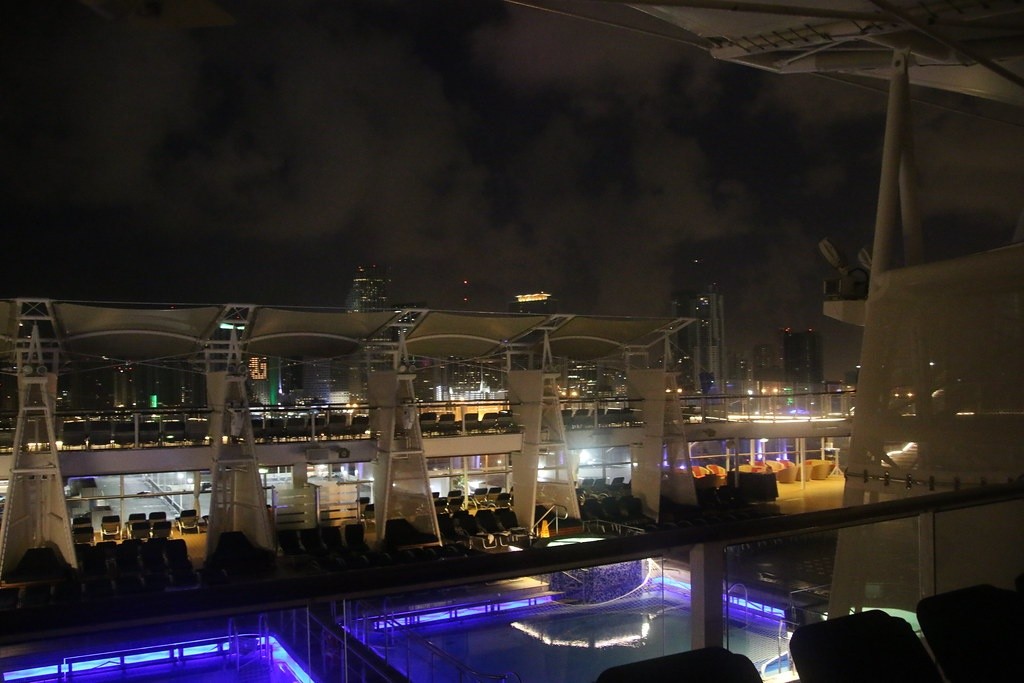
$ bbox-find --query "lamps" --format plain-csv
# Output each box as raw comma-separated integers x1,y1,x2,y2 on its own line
819,237,853,276
23,364,48,375
398,365,417,374
227,364,249,376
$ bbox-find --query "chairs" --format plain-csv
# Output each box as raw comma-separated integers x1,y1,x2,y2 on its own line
561,407,634,430
418,409,512,439
596,575,1024,683
0,459,836,637
60,416,187,448
251,415,372,443
680,405,698,422
